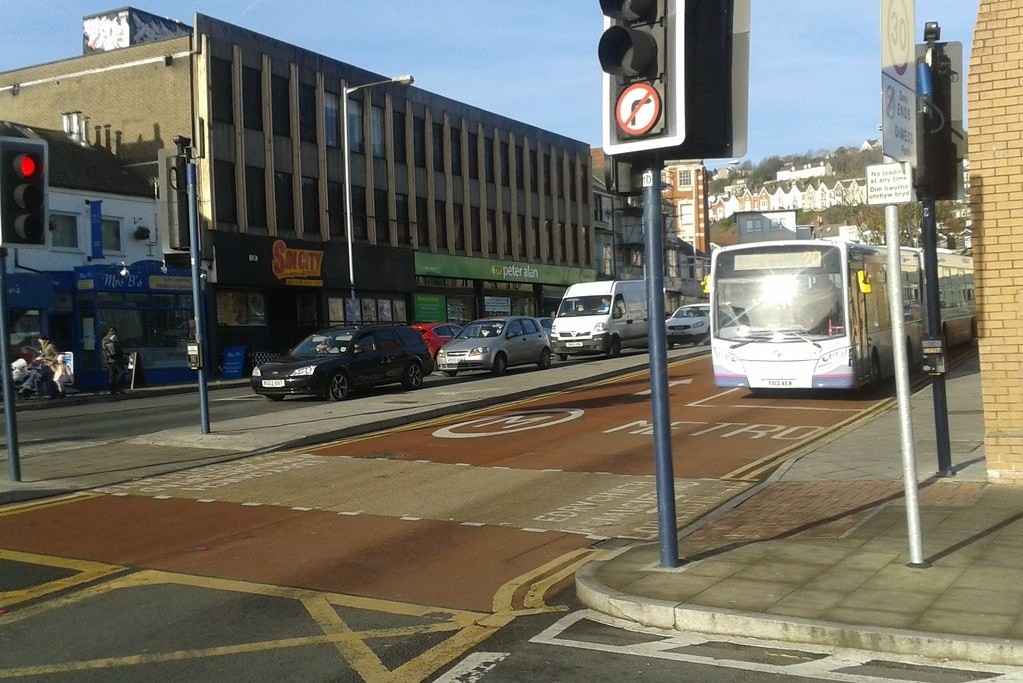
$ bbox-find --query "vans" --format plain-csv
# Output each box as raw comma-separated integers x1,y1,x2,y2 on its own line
550,279,651,362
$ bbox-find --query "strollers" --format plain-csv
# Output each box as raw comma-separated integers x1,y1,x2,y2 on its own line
13,357,54,402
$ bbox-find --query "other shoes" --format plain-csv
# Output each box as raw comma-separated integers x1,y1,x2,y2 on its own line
111,390,125,395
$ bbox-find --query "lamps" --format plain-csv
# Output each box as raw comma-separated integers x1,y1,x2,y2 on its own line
120,262,130,276
160,260,167,274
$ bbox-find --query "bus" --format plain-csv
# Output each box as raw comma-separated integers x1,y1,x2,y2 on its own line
706,235,976,400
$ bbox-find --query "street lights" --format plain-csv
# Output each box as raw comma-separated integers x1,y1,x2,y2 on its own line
339,75,416,301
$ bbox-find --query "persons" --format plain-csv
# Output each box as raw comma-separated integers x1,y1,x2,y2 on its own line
598,298,611,310
705,311,709,316
101,327,127,394
688,311,694,317
38,335,74,398
575,302,587,311
354,340,363,353
324,339,339,353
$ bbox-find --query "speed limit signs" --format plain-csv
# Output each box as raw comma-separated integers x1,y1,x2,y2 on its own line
880,0,916,91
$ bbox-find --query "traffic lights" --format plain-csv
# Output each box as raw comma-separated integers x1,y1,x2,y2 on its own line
0,135,52,252
597,0,692,157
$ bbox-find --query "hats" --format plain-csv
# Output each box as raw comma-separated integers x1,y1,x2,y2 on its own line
109,328,117,331
57,355,65,361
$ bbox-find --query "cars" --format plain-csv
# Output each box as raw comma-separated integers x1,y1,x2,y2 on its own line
435,315,550,378
665,304,712,349
535,316,554,340
410,323,470,360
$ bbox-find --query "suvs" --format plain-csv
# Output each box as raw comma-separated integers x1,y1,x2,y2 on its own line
250,320,434,403
